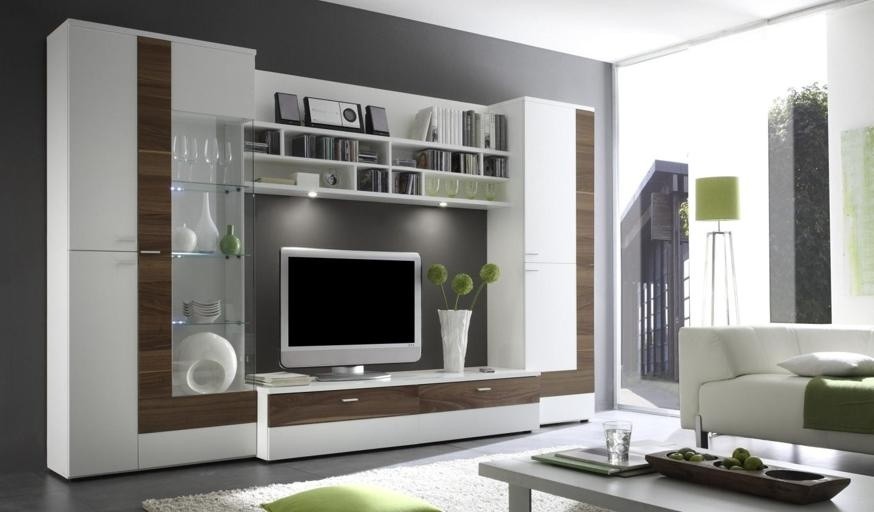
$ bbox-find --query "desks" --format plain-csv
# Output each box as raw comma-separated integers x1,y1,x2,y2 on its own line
478,441,873,511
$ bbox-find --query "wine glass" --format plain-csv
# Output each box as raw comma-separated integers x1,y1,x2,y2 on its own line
171,135,233,185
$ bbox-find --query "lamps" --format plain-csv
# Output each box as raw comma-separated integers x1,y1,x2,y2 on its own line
694,176,740,326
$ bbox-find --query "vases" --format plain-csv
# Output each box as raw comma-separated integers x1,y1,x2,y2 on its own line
194,192,219,252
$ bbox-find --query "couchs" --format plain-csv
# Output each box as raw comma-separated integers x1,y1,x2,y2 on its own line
678,323,873,455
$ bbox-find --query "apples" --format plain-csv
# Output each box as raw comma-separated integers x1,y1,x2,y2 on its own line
689,454,704,462
730,465,744,471
732,447,750,463
717,464,728,471
668,453,683,460
721,456,742,469
702,453,715,460
744,455,764,471
684,451,695,460
679,458,687,461
678,446,694,455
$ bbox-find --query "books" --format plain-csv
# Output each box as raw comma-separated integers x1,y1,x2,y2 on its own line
247,370,312,387
358,170,418,194
247,130,280,154
414,105,508,177
293,133,378,161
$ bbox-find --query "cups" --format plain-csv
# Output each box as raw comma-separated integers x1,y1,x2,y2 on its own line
602,420,632,467
463,179,479,200
426,175,440,196
483,181,498,201
444,177,461,197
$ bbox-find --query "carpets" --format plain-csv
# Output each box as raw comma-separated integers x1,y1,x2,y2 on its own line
141,424,688,512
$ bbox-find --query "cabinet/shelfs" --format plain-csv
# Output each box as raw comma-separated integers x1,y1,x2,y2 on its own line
47,17,595,481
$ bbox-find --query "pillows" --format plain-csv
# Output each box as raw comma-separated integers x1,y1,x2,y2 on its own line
777,353,873,375
260,482,442,511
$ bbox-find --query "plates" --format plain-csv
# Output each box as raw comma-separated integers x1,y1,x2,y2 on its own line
182,298,222,323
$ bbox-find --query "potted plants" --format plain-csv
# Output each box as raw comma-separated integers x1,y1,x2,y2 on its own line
427,262,501,372
